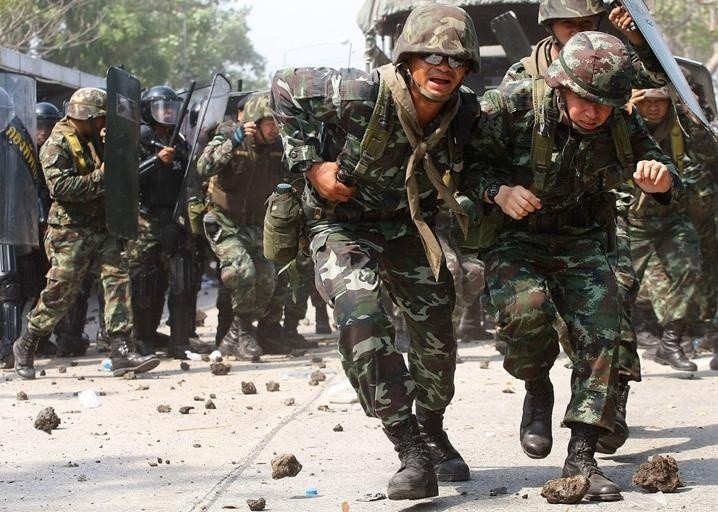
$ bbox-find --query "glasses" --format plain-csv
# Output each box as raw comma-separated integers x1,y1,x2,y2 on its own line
421,53,466,70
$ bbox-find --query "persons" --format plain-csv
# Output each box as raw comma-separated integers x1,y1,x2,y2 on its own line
36,85,331,359
500,1,670,454
270,2,480,499
459,29,687,499
622,66,718,371
11,86,160,376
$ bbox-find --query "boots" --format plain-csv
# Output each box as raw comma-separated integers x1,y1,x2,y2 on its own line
562,421,621,502
216,312,318,359
97,326,211,358
456,303,495,340
520,375,554,459
316,304,331,334
382,414,438,500
110,336,160,376
632,308,717,371
416,404,470,481
36,316,90,357
13,326,42,380
595,379,630,454
394,313,411,352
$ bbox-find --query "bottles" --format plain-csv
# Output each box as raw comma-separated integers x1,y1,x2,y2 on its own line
263,184,298,263
186,196,204,237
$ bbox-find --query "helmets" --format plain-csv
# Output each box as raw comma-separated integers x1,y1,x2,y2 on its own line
34,102,62,134
66,89,109,121
241,89,277,125
538,0,607,27
393,2,483,75
545,30,632,108
641,87,672,100
140,86,183,127
